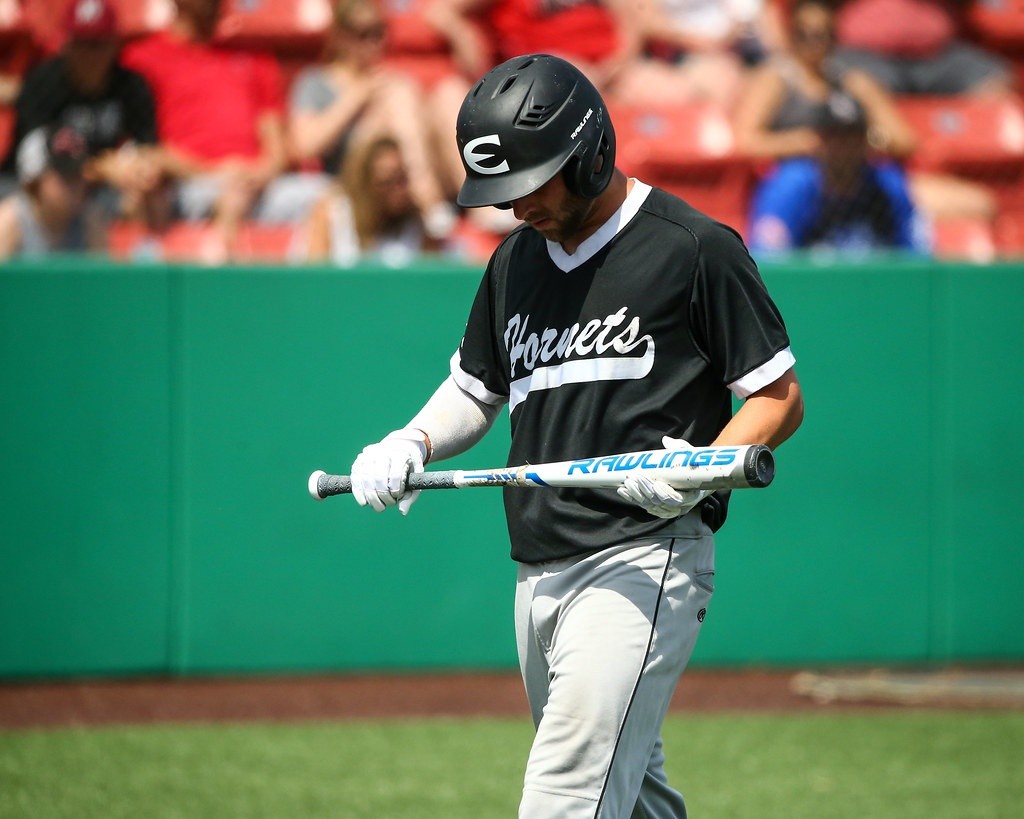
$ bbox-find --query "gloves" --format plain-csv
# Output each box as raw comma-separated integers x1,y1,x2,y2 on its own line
617,435,717,519
350,429,427,515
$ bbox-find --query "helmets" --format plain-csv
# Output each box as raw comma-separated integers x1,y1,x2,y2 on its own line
456,52,615,209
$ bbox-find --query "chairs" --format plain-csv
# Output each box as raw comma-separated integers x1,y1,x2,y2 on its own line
0,0,1024,269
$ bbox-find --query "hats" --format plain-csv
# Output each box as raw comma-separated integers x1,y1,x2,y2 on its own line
62,1,117,51
812,91,867,141
11,124,98,187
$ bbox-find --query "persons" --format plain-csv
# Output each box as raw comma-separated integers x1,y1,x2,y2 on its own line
0,0,360,265
753,93,930,256
743,0,918,157
425,0,617,90
289,0,527,269
354,54,805,819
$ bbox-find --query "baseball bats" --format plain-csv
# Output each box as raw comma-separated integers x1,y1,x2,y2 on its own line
307,444,776,502
